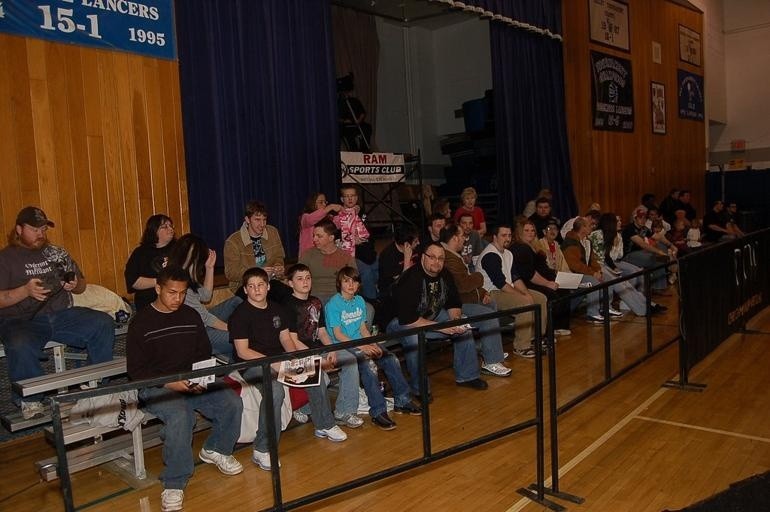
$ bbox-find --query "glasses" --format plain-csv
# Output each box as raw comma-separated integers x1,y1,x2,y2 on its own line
160,223,175,231
544,227,559,232
728,206,737,208
423,252,447,262
22,224,48,233
340,194,357,198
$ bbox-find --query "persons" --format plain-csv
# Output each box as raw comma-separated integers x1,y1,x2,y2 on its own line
228,267,347,471
126,266,243,510
325,267,422,430
283,264,364,428
299,185,379,306
225,202,286,295
0,205,116,422
562,190,747,322
378,184,575,405
125,214,243,353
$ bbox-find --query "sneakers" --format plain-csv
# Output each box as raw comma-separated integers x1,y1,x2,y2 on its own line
291,408,308,422
199,447,243,475
481,329,572,376
160,488,184,511
251,450,282,470
21,400,45,421
315,425,347,442
334,413,364,429
586,302,669,324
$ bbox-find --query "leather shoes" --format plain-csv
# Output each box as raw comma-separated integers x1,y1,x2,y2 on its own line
371,411,396,430
394,400,422,416
457,378,485,390
416,392,432,402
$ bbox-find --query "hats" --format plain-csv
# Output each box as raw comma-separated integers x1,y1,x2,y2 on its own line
16,206,56,228
633,208,648,217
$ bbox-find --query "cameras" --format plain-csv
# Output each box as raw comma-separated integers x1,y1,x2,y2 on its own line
46,267,75,281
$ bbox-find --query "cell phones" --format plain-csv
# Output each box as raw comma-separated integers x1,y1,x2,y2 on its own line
188,382,199,389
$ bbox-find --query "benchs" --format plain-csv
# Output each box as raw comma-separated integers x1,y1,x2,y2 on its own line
1,264,513,481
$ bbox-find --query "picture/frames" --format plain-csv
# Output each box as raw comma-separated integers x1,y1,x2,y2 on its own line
588,0,632,55
678,22,705,69
649,77,667,136
